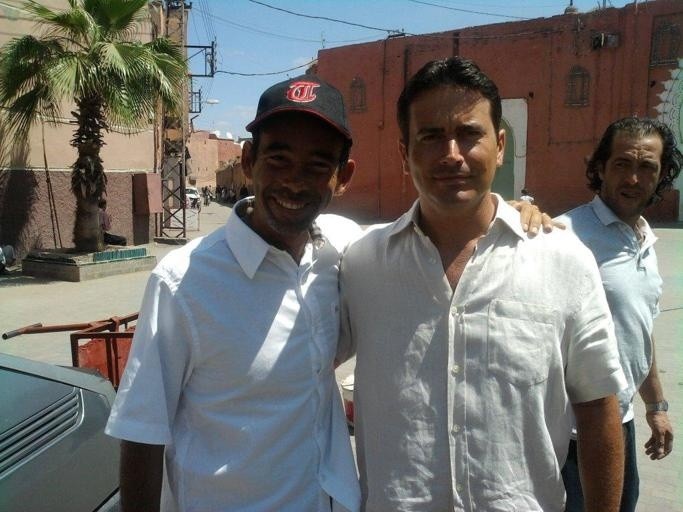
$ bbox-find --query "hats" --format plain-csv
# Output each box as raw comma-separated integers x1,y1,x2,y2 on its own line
246,74,352,150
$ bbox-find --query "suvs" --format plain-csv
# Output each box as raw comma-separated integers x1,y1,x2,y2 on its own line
185,187,201,204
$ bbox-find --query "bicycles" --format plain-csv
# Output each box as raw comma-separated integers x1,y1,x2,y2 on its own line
206,193,211,206
190,197,201,214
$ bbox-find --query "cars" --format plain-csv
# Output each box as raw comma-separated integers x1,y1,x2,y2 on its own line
0,345,128,512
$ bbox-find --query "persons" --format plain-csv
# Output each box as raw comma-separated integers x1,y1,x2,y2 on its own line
98,198,126,246
102,72,568,511
333,54,628,510
199,185,249,205
518,186,536,204
550,115,681,512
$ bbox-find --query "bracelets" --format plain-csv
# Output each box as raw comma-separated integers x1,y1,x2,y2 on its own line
643,402,667,412
